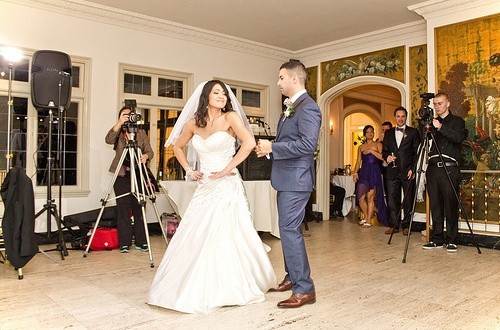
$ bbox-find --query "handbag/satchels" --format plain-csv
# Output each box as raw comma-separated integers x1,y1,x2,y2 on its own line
159,212,180,238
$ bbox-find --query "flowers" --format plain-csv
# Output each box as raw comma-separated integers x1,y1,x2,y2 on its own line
326,53,401,82
282,98,297,122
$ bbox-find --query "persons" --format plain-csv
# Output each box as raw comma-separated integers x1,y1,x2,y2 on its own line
104,106,154,254
417,92,466,253
328,181,346,219
255,60,323,309
146,80,278,315
352,105,420,236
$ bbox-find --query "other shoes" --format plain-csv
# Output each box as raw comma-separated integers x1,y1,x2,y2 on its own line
120,245,129,253
403,228,411,235
135,244,149,252
446,243,458,252
422,241,443,249
385,228,399,234
331,209,344,219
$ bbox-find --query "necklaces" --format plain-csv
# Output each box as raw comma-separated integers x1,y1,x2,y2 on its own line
366,143,375,149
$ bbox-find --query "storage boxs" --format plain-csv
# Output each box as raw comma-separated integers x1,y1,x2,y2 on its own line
88,227,119,251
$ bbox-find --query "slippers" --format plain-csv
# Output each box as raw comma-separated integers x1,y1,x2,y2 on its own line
358,219,367,226
362,223,372,228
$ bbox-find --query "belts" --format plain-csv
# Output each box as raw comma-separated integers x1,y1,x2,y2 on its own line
437,161,457,168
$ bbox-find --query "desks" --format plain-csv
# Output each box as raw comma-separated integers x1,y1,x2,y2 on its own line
333,175,355,216
159,180,282,239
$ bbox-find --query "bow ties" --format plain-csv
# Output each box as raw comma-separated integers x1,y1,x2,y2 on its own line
396,127,404,132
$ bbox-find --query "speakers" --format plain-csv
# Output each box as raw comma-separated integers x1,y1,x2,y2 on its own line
237,136,276,181
30,50,72,110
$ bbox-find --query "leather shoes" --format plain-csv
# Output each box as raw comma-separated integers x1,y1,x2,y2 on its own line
271,280,292,292
276,292,316,308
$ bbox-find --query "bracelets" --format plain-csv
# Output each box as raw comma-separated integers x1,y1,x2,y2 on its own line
352,173,358,176
185,166,194,182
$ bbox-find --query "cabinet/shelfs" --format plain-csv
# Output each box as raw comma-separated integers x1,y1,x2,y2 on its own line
238,136,275,180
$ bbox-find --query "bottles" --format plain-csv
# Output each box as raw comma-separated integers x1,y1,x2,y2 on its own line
345,166,351,176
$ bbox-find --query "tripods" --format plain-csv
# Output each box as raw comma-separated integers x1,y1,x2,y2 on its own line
387,127,482,262
6,68,168,281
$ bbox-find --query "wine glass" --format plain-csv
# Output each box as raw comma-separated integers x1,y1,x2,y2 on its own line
390,152,397,168
